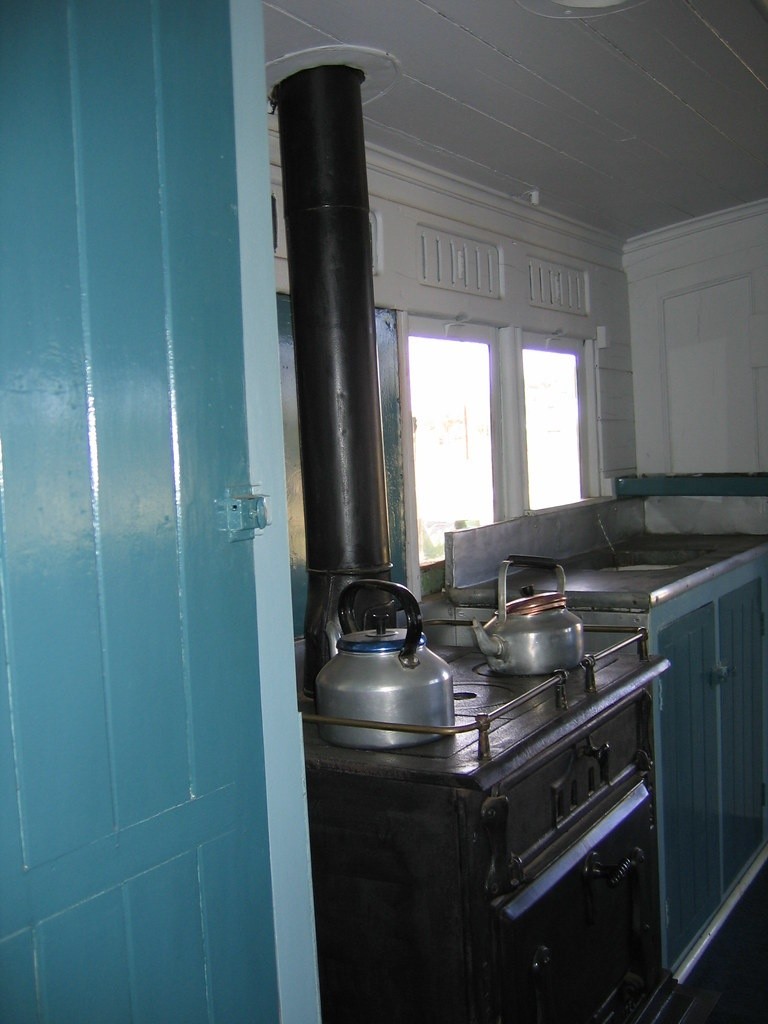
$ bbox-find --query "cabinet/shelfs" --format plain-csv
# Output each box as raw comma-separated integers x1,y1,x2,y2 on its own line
456,554,768,984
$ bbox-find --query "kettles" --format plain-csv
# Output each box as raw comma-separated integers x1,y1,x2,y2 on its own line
470,554,586,676
315,579,455,749
